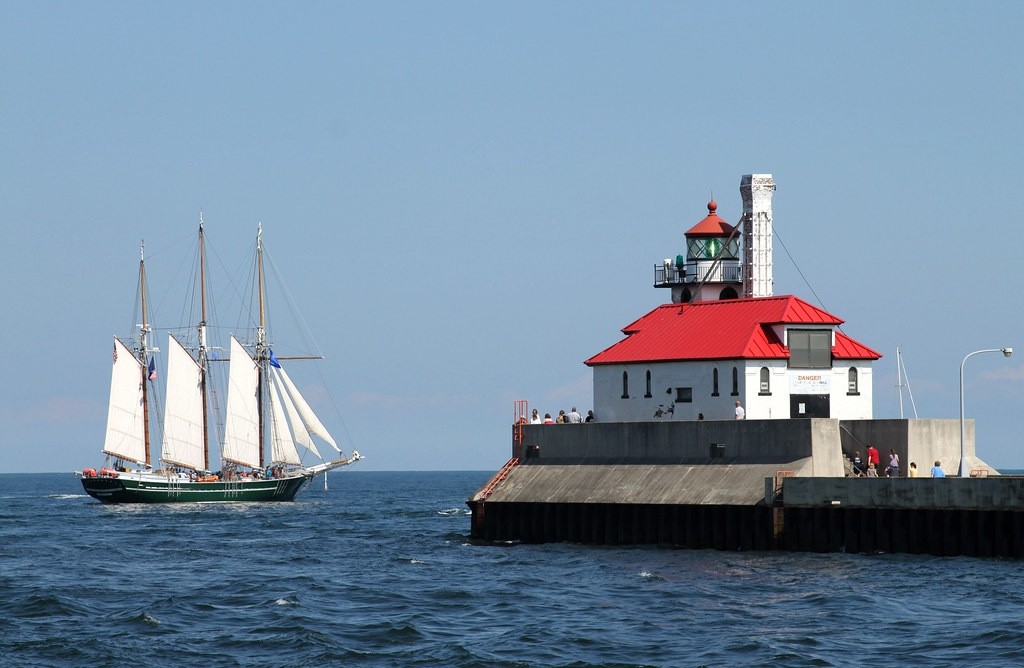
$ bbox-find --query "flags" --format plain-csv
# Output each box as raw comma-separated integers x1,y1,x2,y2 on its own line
148,356,157,381
270,349,281,369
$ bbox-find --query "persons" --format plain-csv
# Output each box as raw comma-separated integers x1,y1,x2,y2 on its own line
866,463,878,477
530,408,541,424
516,415,525,424
556,407,583,423
190,470,200,482
931,461,945,477
697,413,704,420
866,444,880,470
735,401,744,419
585,410,594,422
243,463,284,480
851,451,866,477
885,449,901,477
910,462,918,477
543,414,553,424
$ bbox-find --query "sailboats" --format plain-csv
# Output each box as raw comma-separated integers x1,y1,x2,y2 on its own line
75,209,364,503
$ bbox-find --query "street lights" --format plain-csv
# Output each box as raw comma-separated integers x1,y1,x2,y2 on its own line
959,348,1012,478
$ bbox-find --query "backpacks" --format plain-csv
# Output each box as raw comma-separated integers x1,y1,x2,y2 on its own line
563,415,569,423
